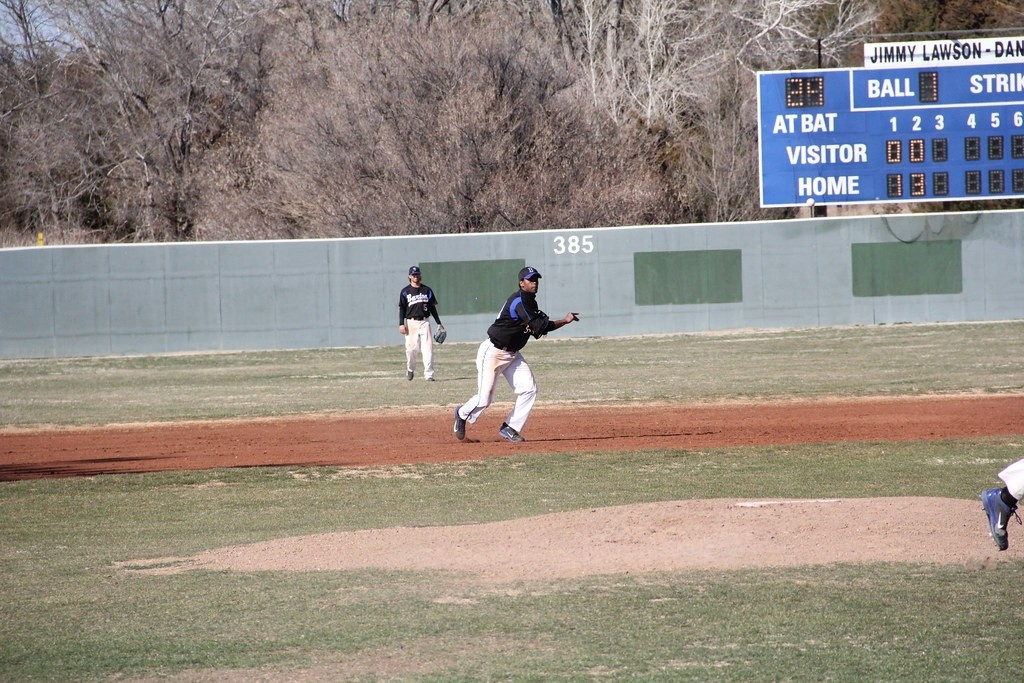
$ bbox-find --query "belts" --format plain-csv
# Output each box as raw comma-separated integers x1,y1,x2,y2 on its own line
491,340,515,352
407,316,424,320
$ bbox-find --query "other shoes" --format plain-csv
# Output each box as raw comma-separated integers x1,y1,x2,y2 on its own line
406,371,413,381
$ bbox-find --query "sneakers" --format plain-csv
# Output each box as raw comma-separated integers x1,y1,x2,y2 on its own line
454,406,466,440
981,488,1021,550
498,422,525,442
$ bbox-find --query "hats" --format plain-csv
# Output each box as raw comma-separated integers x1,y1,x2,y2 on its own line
409,266,421,275
518,266,542,280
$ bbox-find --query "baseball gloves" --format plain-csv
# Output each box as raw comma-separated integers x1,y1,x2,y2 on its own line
433,325,447,344
530,310,549,339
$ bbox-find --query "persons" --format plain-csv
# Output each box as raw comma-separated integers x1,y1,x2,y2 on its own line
453,267,580,443
398,266,446,381
980,457,1024,551
